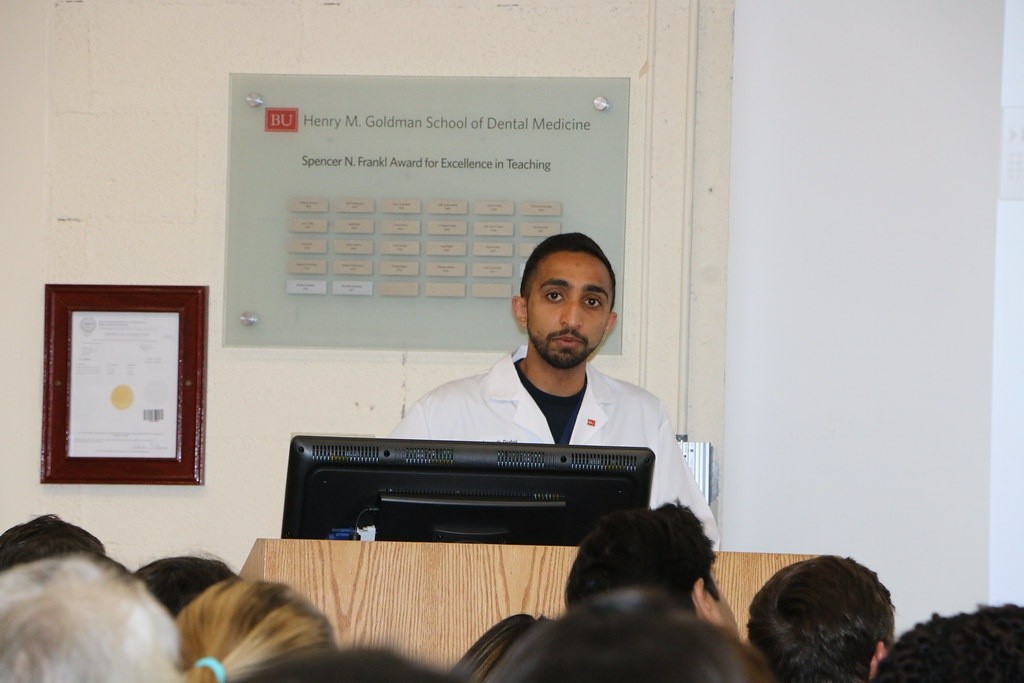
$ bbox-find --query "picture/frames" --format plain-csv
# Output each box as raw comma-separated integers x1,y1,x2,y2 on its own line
39,283,212,485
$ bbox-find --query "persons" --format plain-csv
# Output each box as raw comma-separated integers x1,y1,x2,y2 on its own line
876,603,1024,683
563,502,739,640
0,513,105,574
0,555,183,683
745,558,897,683
233,647,465,683
386,233,721,550
448,615,544,683
485,599,777,683
132,555,240,622
172,576,336,683
